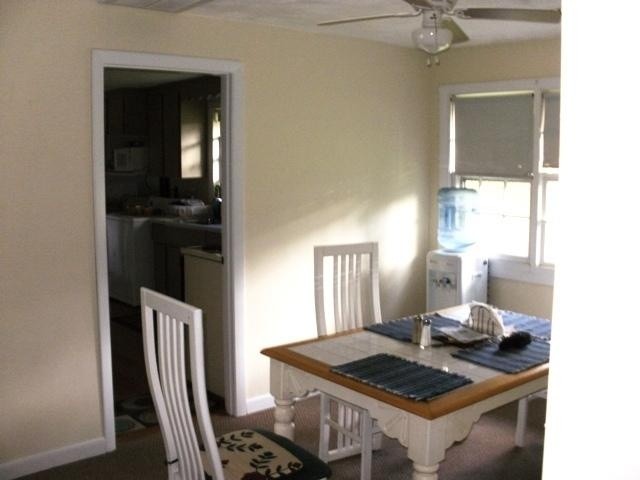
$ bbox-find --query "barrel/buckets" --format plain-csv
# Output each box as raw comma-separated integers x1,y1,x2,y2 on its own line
435,187,481,253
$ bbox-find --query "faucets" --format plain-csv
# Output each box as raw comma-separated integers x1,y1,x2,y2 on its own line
215,186,220,198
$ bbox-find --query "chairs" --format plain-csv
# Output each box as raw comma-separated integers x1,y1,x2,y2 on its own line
140,287,332,480
314,242,383,480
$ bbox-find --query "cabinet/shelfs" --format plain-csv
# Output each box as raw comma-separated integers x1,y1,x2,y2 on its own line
107,94,146,137
146,93,205,180
150,222,206,301
107,217,150,306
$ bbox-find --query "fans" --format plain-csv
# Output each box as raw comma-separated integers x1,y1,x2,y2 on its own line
317,0,561,43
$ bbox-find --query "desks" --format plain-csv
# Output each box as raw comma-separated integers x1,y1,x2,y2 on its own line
260,303,551,480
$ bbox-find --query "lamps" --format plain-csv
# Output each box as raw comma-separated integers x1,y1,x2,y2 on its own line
411,10,454,66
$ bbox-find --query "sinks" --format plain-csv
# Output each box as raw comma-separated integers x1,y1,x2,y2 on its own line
170,217,220,226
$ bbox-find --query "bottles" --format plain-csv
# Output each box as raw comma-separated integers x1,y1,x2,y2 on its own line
419,319,432,350
412,314,423,345
